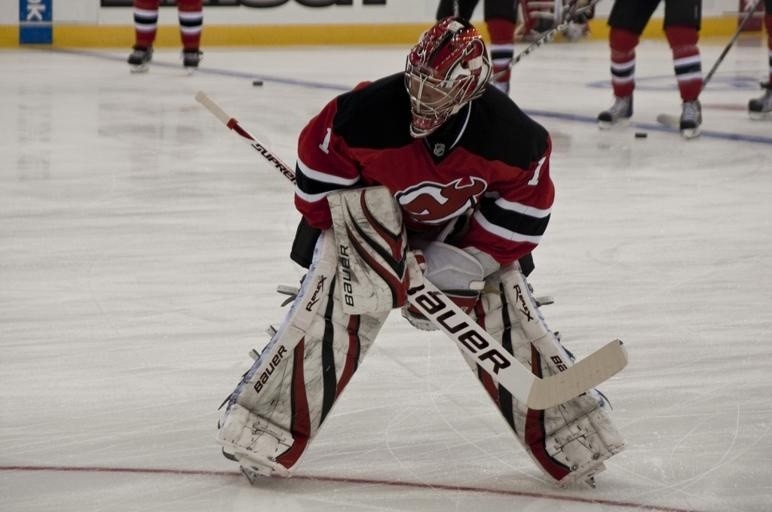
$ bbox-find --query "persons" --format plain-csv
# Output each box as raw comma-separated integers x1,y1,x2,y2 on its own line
746,2,772,115
218,17,615,491
598,2,705,131
435,1,552,99
125,2,204,67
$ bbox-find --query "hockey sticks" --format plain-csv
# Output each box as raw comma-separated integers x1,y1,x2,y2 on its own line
195,89,628,411
657,0,760,127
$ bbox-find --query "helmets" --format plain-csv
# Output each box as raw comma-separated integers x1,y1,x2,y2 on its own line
402,17,494,139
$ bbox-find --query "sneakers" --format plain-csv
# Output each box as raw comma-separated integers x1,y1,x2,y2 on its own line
749,88,772,112
128,47,152,64
597,95,633,120
184,50,199,66
680,100,702,129
